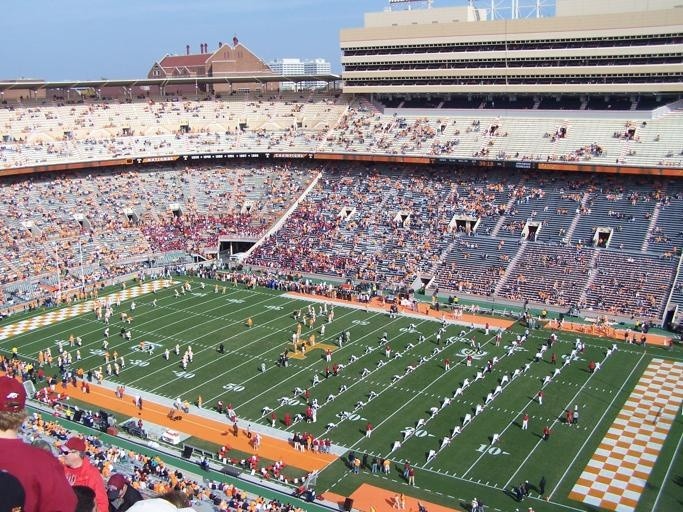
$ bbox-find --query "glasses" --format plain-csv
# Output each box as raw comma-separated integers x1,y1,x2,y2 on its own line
64,451,76,455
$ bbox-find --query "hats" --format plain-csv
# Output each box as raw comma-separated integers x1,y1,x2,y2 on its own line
107,473,125,501
0,471,25,511
57,438,86,452
0,376,26,412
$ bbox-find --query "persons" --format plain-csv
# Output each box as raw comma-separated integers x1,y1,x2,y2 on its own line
0,88,683,512
